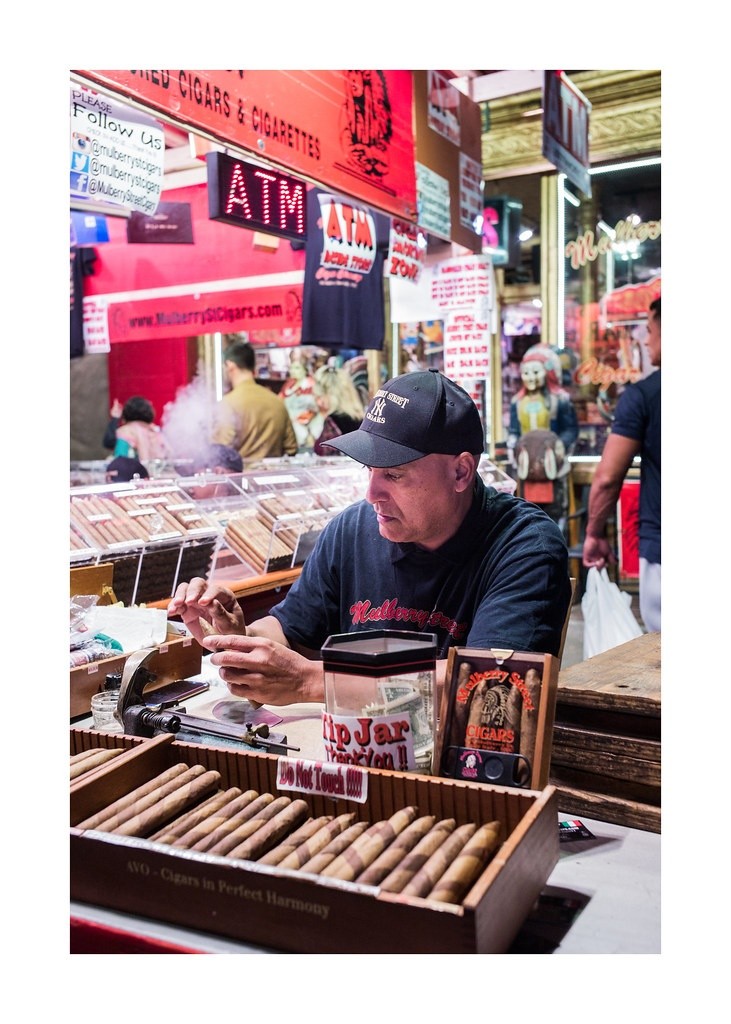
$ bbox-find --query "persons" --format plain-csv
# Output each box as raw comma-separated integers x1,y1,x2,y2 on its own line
167,367,573,705
505,342,579,548
102,338,365,472
583,298,661,633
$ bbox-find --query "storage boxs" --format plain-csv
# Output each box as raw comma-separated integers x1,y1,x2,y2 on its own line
71,631,203,722
70,726,568,954
70,452,518,607
548,632,661,835
433,646,561,792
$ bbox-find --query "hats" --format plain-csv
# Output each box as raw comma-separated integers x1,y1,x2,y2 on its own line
318,368,485,468
173,443,243,477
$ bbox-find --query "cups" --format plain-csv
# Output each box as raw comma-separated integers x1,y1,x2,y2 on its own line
90,691,124,734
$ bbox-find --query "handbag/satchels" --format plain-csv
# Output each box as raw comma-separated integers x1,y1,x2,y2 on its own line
580,568,645,660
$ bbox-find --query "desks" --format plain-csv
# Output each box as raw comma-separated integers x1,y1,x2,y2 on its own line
70,810,661,954
